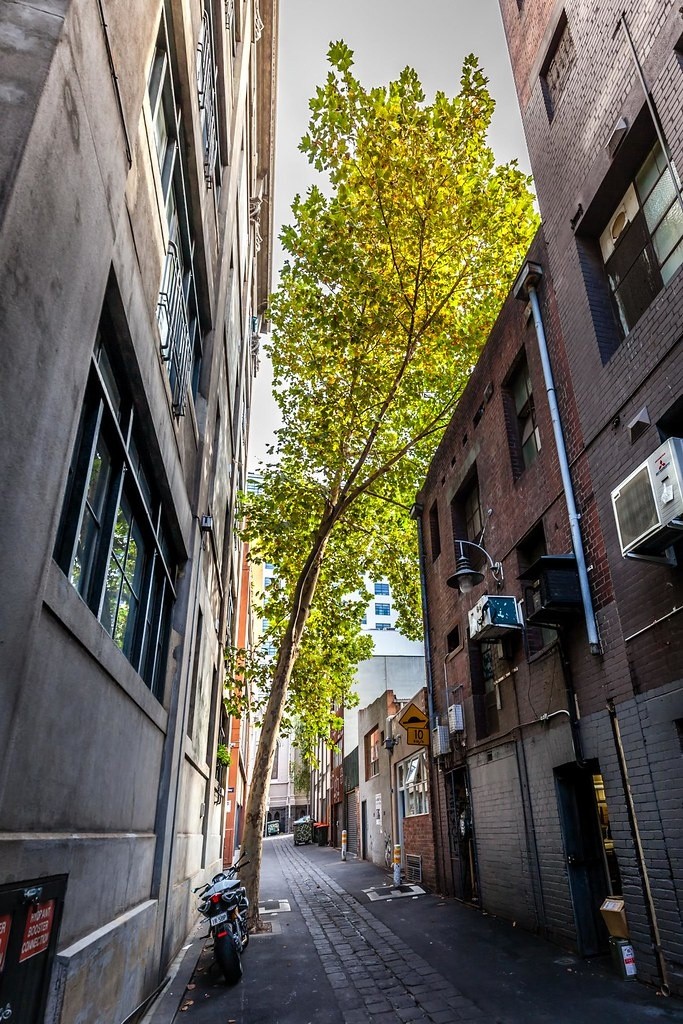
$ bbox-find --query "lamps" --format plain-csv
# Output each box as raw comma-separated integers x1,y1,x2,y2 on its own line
445,539,500,593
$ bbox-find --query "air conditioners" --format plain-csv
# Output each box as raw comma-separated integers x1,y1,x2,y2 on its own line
608,437,683,563
467,595,519,638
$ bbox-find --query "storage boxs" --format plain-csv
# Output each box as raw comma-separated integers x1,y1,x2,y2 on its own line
599,896,630,940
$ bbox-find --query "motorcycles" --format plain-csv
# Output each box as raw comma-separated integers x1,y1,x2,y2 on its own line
191,850,259,983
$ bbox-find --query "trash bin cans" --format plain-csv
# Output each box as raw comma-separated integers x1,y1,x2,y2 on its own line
267,820,279,836
313,823,329,846
293,820,313,845
